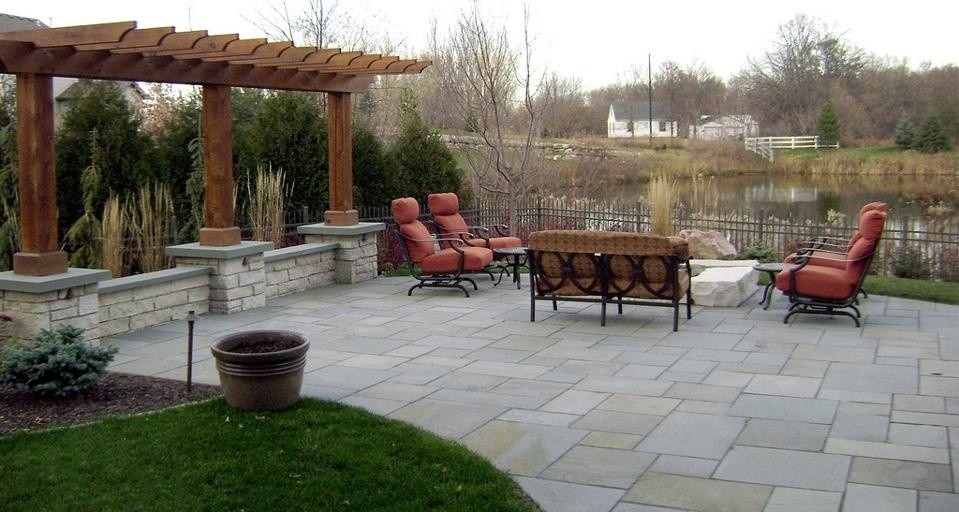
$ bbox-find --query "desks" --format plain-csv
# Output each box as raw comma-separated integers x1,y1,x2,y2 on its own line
752,263,784,310
493,246,528,290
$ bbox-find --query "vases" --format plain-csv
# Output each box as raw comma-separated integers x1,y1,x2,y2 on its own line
211,329,309,410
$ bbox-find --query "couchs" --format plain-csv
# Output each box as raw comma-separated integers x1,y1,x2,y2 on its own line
524,230,692,332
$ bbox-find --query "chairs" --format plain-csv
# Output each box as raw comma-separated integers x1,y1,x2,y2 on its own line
428,192,521,280
777,201,887,327
391,197,493,299
